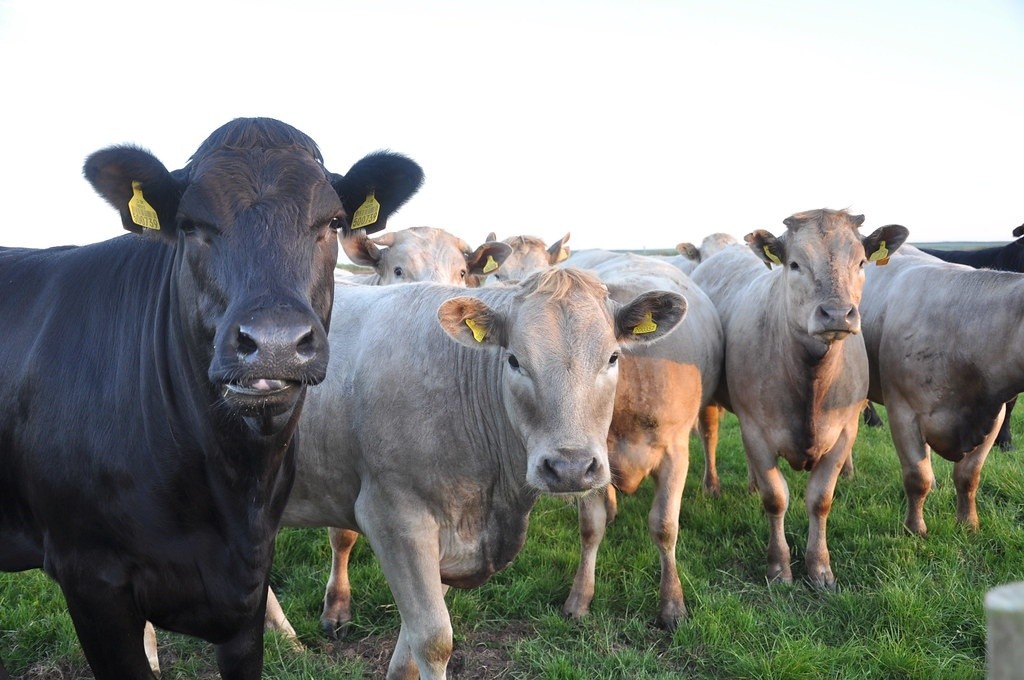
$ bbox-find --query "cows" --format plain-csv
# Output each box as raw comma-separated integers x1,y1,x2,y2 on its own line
0,114,1023,678
